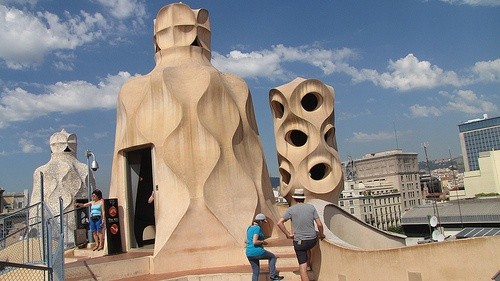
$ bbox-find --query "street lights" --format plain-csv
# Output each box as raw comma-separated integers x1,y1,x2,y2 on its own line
85,149,99,249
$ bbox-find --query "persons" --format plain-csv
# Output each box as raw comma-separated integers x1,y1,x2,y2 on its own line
244,213,284,281
147,191,155,217
76,189,105,251
277,189,325,281
19,221,29,241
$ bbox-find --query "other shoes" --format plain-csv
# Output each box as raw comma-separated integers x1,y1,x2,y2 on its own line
92,245,104,251
270,275,284,280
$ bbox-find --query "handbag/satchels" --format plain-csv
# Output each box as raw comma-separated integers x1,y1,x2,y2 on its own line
91,216,100,222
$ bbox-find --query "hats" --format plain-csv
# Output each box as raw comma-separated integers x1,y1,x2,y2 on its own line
292,189,306,199
255,213,268,223
